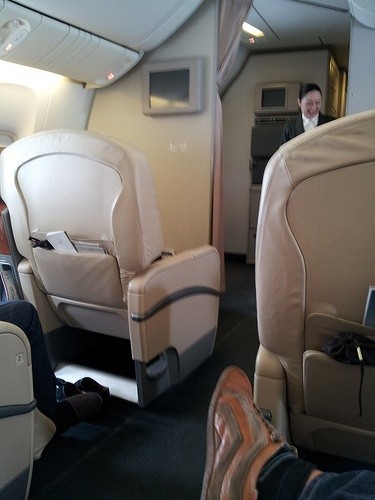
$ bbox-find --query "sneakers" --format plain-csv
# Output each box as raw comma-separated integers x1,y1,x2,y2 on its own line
199,367,298,500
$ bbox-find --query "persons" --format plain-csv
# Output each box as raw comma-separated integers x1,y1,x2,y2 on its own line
200,365,375,500
280,83,337,148
1,299,103,428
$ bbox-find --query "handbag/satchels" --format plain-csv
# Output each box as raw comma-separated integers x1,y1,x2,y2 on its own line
319,331,375,418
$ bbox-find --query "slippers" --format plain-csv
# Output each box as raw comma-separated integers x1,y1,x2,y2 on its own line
56,377,111,404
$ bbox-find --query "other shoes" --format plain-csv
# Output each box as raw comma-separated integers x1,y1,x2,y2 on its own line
58,392,103,433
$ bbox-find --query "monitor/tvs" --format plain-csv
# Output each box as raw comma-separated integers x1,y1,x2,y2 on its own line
258,85,289,112
142,58,203,116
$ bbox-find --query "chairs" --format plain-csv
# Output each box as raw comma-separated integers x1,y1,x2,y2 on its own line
0,317,57,500
0,127,221,411
243,106,375,472
